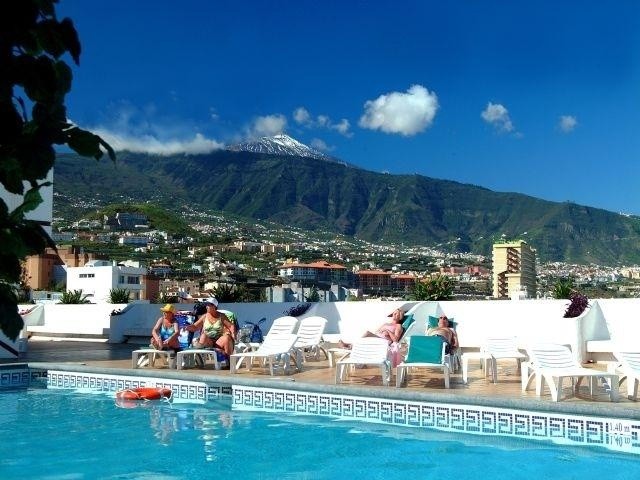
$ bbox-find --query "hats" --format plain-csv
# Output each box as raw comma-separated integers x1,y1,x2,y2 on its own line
203,298,219,310
161,304,175,314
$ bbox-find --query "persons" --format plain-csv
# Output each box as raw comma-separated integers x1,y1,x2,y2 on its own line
193,405,232,464
185,297,238,367
340,308,406,351
424,316,459,355
150,304,178,359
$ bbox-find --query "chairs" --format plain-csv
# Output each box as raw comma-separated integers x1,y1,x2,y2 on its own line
134,308,329,379
335,307,639,407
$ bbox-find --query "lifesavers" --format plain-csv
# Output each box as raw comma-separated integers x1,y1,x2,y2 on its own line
117,388,170,400
